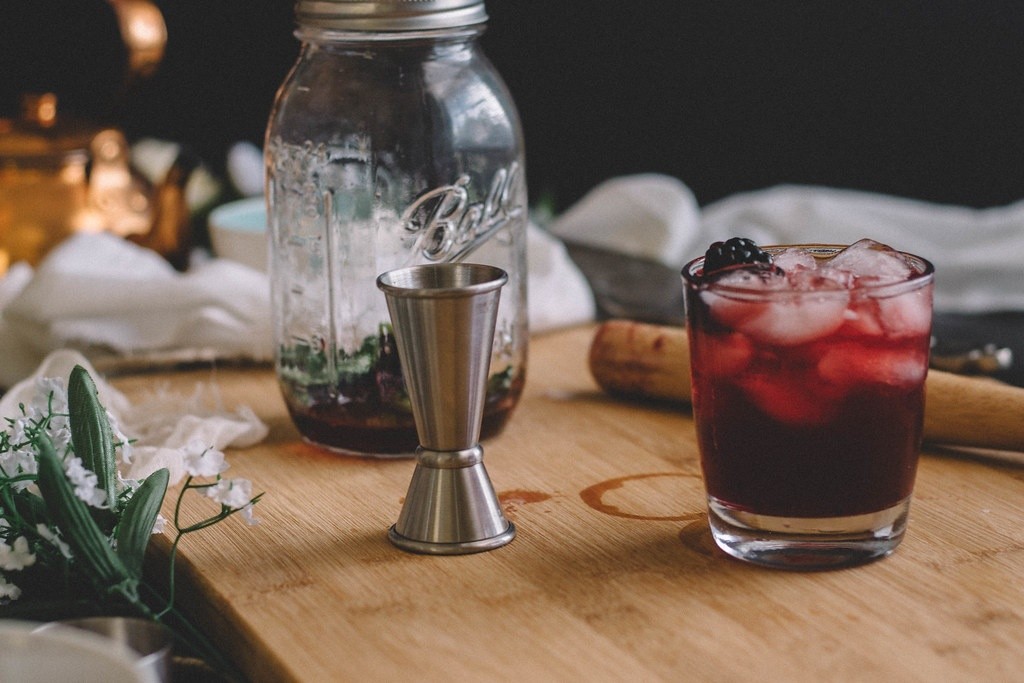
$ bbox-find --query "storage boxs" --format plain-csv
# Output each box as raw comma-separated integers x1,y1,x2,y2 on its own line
263,0,533,458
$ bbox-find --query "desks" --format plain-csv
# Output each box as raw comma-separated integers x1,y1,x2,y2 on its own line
103,324,1024,683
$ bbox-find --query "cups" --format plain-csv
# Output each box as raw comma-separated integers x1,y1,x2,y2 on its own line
682,244,936,574
374,261,518,558
